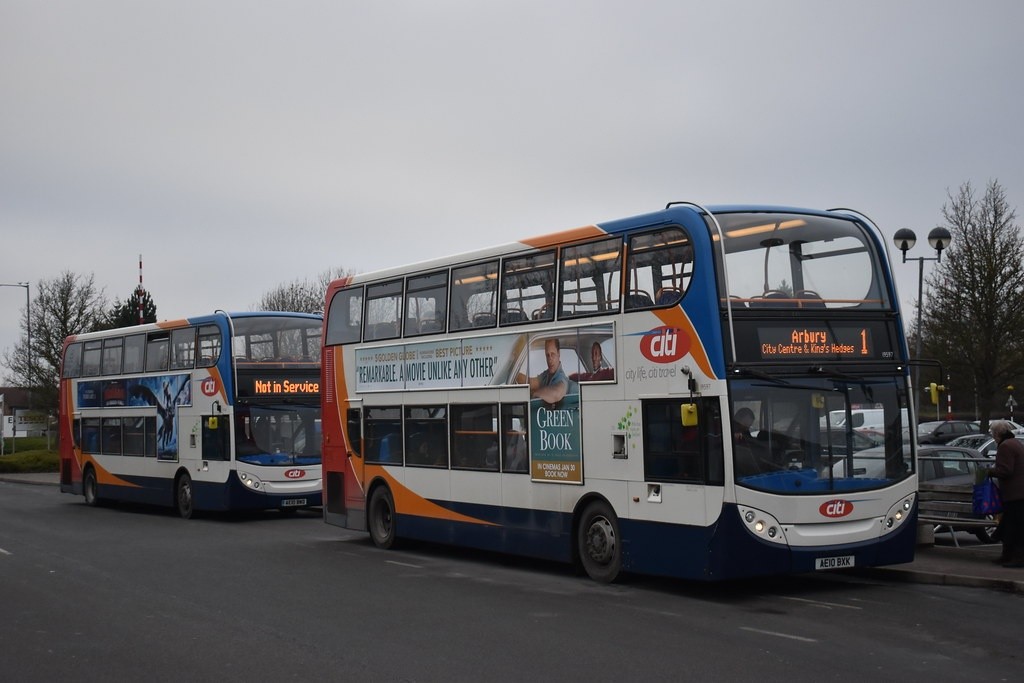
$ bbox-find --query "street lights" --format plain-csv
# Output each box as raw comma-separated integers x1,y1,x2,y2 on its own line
893,227,952,425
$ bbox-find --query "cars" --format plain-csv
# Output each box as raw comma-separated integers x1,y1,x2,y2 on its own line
750,417,1024,543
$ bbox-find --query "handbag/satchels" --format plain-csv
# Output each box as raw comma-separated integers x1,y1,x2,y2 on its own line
972,474,1006,514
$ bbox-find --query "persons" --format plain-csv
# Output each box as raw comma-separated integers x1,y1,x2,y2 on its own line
986,421,1024,567
515,340,569,403
570,342,615,382
679,407,774,478
403,425,528,471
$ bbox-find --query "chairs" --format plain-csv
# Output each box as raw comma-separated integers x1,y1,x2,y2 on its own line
66,356,314,376
654,286,685,306
725,290,827,307
351,304,562,341
375,431,484,471
75,429,110,454
630,289,654,307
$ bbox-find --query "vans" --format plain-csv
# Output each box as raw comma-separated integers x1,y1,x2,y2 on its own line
823,408,909,428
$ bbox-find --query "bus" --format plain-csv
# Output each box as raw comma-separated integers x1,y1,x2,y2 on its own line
59,309,324,519
322,202,946,583
